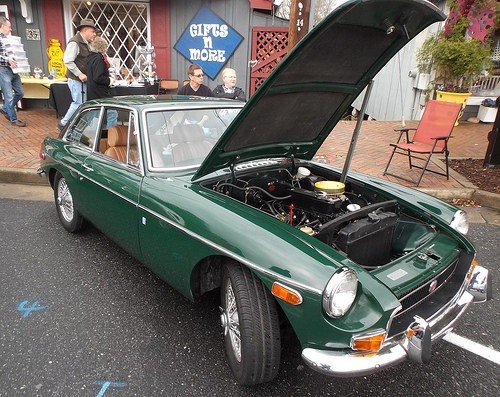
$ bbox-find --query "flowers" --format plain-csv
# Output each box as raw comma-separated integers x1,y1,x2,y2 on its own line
481,98,496,107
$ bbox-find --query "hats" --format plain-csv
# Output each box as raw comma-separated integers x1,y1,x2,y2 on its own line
76,18,96,31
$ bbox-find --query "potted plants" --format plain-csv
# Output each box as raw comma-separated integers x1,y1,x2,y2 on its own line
417,15,496,127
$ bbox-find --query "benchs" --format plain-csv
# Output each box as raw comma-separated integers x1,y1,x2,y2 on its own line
87,138,110,154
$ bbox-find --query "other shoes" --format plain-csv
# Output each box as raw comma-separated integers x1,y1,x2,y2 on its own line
0,108,11,121
57,122,65,130
12,119,26,127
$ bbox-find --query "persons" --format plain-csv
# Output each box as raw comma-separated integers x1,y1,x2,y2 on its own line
213,68,247,138
86,36,118,128
176,64,213,136
57,19,97,130
0,15,26,126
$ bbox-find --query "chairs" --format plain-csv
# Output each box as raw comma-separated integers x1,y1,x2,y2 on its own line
171,124,213,167
103,126,161,162
158,79,179,95
383,100,463,190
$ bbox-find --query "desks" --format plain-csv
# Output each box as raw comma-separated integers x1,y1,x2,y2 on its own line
20,76,67,118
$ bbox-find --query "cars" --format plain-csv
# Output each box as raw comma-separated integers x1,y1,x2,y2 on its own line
38,0,488,388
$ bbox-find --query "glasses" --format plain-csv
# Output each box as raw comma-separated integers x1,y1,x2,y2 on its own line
193,74,204,77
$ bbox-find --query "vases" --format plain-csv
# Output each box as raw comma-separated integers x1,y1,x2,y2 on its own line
477,105,498,123
